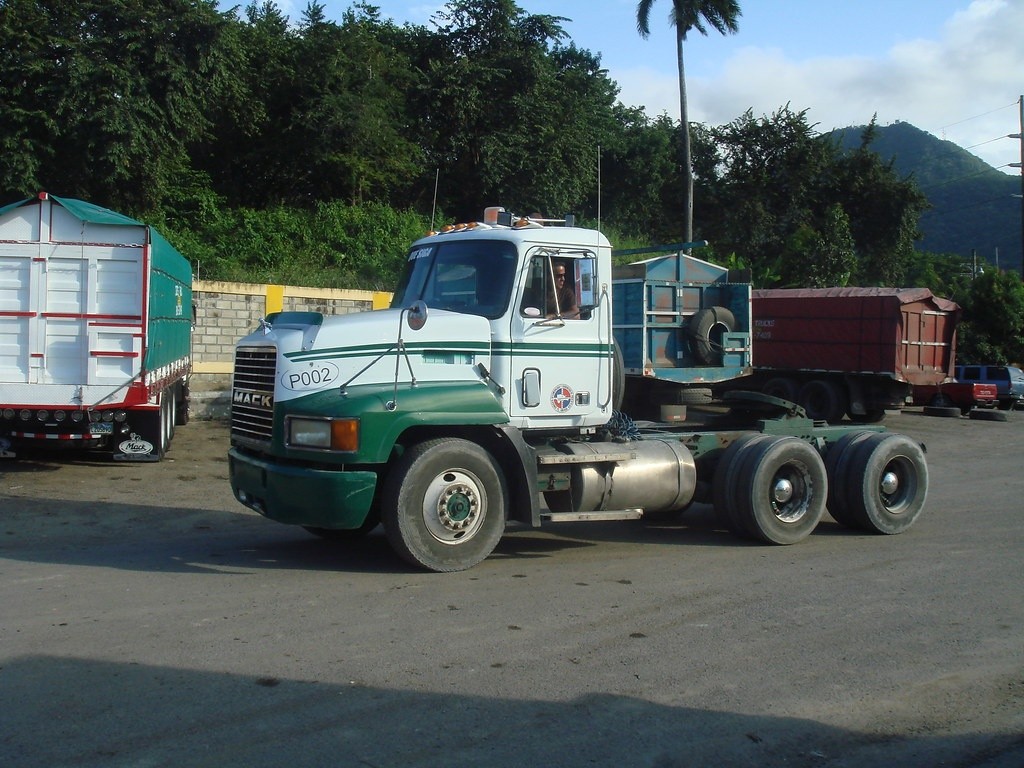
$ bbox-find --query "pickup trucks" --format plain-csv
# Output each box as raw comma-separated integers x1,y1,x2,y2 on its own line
913,377,999,416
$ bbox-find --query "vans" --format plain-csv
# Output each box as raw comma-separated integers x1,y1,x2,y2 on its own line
955,365,1024,410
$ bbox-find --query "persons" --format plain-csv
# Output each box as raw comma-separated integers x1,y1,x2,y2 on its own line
530,262,580,320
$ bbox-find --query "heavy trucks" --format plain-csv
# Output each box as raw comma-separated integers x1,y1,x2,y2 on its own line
753,287,963,423
226,205,932,574
1,193,198,462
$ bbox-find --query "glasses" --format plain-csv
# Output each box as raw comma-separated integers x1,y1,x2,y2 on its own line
553,273,567,280
